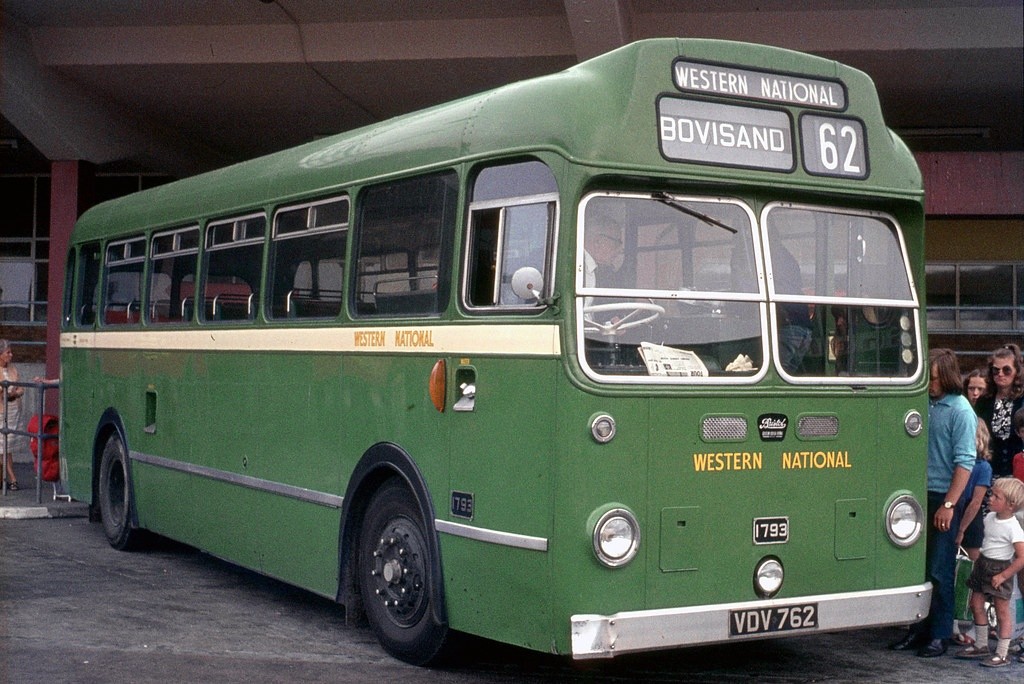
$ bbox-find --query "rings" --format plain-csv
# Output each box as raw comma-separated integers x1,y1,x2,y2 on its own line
941,524,945,527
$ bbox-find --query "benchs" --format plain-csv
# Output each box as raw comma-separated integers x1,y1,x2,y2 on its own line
81,273,440,324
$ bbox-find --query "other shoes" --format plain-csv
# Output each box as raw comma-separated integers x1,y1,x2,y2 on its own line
9,482,18,491
955,643,990,658
993,634,1024,663
978,653,1011,667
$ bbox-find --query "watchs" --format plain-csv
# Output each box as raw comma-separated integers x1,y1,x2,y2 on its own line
942,502,955,509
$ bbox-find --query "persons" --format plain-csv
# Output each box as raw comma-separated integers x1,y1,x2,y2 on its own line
889,348,979,657
974,344,1024,642
954,478,1024,668
584,212,635,318
832,261,908,378
963,368,988,407
1008,407,1024,663
33,377,60,384
953,418,992,646
728,209,812,376
0,339,25,491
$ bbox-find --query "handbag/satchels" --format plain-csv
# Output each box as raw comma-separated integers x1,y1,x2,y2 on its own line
26,414,61,484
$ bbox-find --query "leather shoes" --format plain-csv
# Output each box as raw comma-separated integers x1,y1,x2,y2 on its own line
917,636,949,656
891,632,931,651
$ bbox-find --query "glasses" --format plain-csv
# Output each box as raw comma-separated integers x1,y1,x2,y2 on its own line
597,232,623,250
991,365,1015,375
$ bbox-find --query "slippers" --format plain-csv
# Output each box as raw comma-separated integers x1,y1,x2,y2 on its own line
952,631,975,645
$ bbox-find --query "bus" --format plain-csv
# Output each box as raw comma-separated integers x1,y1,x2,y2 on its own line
58,37,932,667
